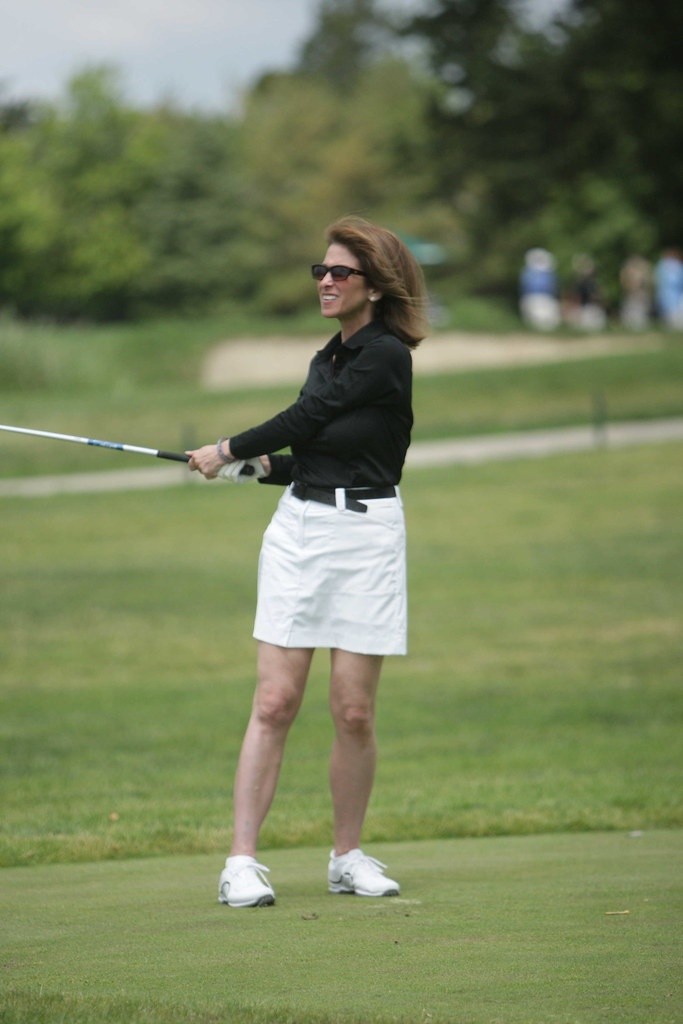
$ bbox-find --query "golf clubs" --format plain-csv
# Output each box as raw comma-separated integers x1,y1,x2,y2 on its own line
1,424,256,477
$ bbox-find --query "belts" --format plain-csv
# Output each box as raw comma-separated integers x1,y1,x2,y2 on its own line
291,481,398,514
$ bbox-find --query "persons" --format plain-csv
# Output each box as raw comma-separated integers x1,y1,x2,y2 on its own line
518,248,563,331
562,248,683,331
184,217,425,906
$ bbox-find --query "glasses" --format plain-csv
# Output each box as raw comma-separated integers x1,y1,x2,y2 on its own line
310,263,368,282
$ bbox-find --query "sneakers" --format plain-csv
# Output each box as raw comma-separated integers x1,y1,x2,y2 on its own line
324,848,401,896
218,861,275,908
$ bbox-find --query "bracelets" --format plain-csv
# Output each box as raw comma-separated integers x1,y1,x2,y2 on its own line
217,437,234,462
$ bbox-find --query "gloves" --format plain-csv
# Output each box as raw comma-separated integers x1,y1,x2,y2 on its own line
216,454,266,483
